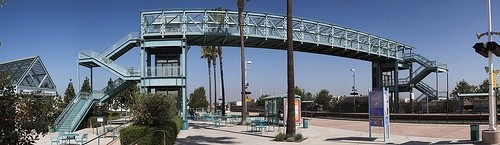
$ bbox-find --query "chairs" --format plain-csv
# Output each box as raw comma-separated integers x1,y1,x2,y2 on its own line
50,125,118,145
190,109,284,136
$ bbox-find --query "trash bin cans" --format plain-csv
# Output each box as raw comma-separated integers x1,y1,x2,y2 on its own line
303,119,308,128
470,124,479,141
181,120,188,130
50,126,54,133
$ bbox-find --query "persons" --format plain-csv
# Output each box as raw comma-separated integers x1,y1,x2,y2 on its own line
277,109,281,119
189,109,196,118
